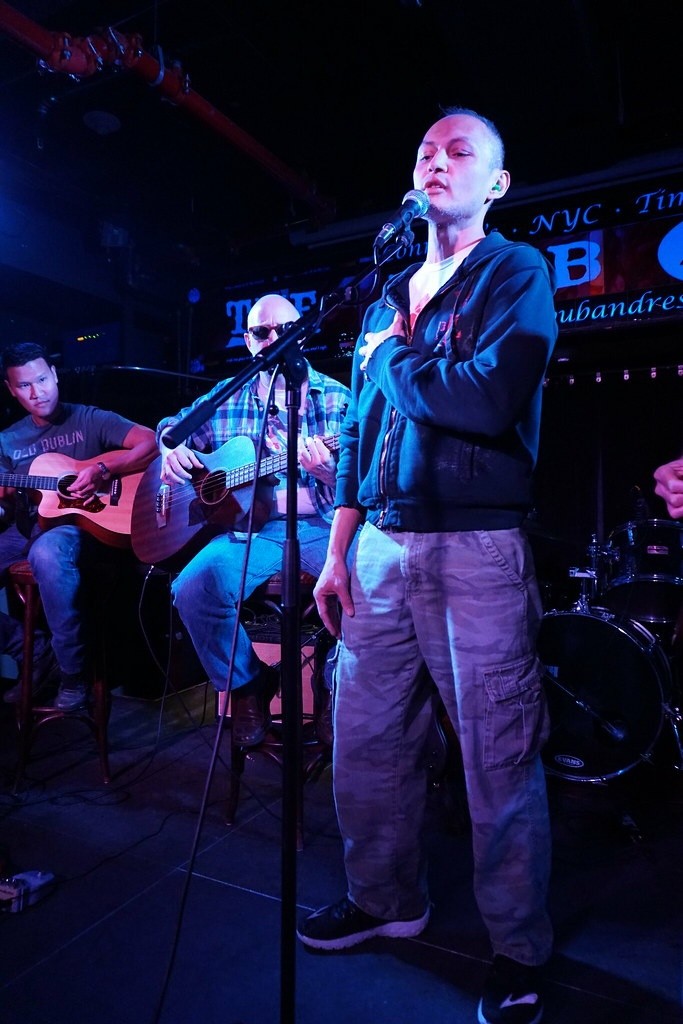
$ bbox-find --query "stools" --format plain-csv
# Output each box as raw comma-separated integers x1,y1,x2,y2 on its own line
5,558,115,797
219,566,343,854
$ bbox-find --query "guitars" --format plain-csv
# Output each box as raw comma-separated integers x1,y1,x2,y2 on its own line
1,449,146,551
128,432,340,575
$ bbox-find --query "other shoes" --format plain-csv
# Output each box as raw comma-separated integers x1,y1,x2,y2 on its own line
3,663,60,703
56,672,87,710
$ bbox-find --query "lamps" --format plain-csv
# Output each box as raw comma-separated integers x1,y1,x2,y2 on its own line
30,23,191,106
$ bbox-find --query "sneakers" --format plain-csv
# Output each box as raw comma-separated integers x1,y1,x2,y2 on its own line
477,953,549,1024
296,892,431,950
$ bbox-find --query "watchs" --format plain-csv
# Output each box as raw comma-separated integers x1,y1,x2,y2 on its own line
96,460,111,480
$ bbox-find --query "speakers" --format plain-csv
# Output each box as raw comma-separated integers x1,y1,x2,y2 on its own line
215,624,337,728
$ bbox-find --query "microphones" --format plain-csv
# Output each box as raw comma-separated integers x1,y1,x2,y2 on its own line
614,720,628,741
633,485,647,521
374,189,431,249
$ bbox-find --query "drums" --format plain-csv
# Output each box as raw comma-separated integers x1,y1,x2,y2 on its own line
597,516,683,625
535,604,675,786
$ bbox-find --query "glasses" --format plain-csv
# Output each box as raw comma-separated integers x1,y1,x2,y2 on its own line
249,321,294,342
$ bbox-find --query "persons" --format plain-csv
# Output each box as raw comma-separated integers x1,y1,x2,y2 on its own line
292,108,558,1024
152,294,363,751
0,342,161,712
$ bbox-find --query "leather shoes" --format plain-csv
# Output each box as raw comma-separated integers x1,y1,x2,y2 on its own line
231,662,280,746
311,669,333,745
